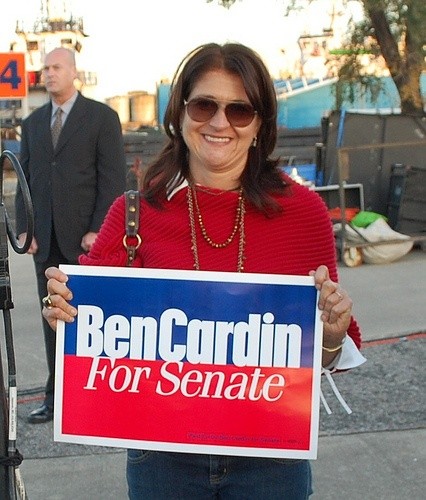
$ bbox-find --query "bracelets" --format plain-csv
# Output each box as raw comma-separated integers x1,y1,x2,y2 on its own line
322,337,346,353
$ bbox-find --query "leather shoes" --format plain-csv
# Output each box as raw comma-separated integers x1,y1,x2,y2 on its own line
28,405,53,423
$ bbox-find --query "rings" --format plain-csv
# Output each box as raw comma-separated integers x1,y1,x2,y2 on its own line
335,291,344,301
42,293,53,310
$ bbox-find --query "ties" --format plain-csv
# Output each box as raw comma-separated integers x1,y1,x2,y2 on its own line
51,108,63,149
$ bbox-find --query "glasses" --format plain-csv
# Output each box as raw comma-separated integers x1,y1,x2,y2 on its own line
182,97,258,127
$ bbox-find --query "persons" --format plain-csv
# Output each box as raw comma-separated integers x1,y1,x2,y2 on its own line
14,45,127,422
39,41,362,500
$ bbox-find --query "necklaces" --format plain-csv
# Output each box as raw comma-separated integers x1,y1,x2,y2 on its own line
186,179,246,272
